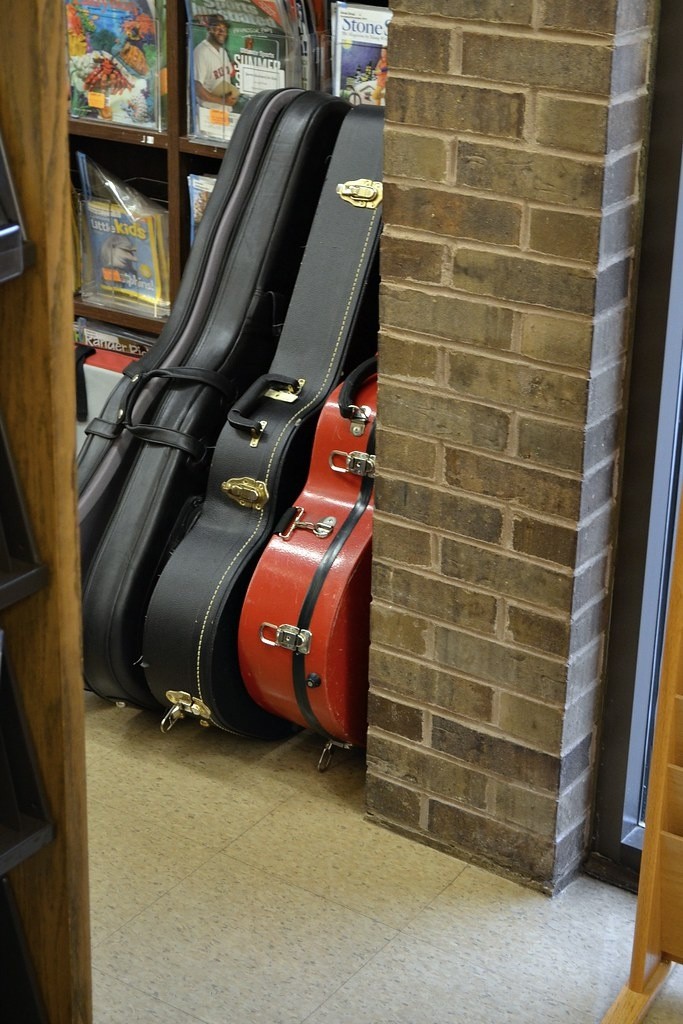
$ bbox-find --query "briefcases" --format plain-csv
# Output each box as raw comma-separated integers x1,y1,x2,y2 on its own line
70,88,353,706
236,339,381,770
143,105,389,742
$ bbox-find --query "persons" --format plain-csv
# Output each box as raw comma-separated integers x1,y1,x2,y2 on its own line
371,47,388,110
191,14,235,119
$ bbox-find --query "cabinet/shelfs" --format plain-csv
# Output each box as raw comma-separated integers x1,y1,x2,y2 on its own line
0,0,92,1024
597,479,682,1023
76,0,391,427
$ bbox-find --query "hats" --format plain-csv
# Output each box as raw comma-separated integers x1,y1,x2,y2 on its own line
203,13,229,28
